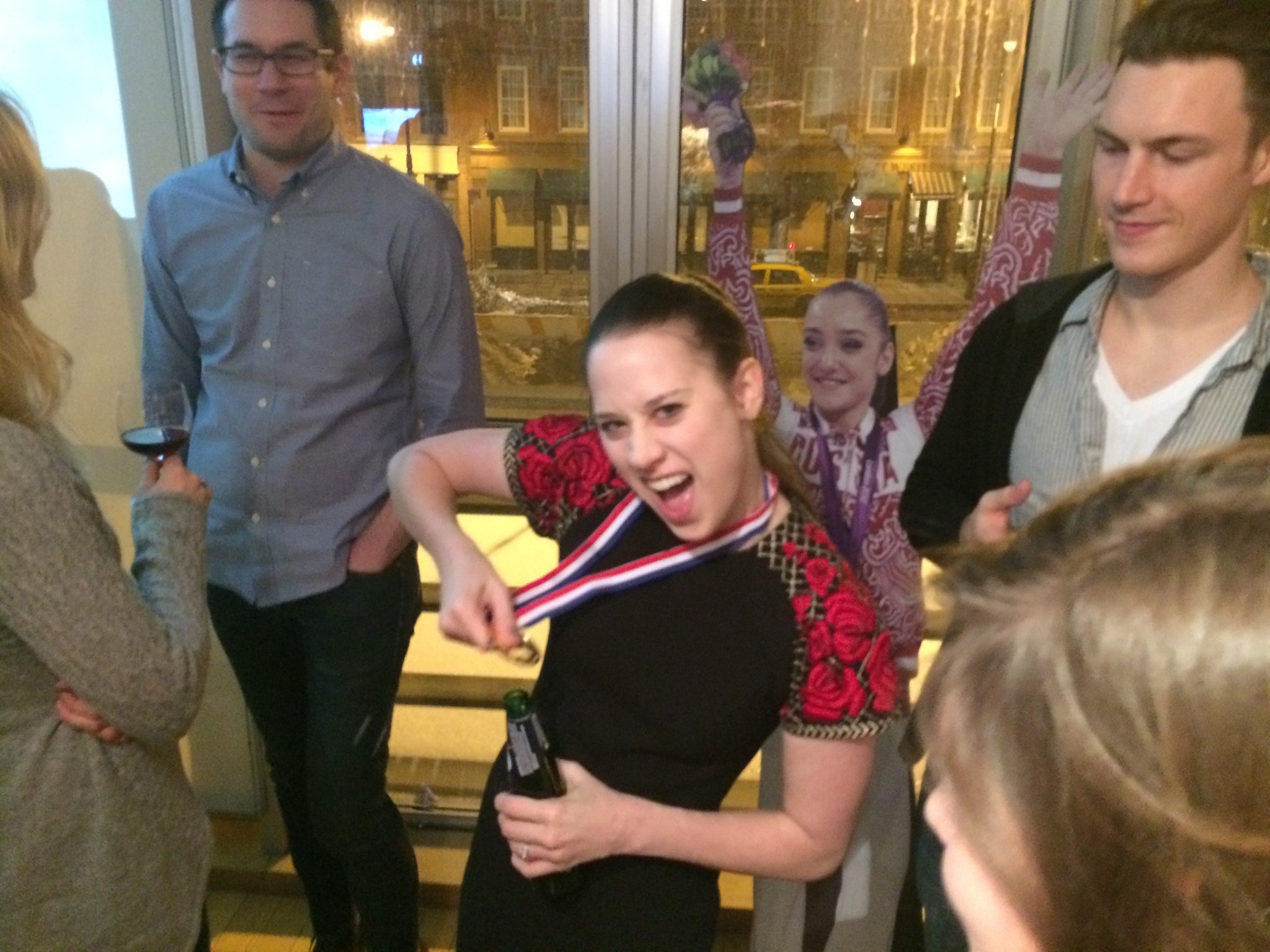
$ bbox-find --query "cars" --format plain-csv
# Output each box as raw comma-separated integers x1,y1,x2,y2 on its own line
747,261,848,299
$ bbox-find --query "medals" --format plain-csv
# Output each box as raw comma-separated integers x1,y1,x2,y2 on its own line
496,639,542,669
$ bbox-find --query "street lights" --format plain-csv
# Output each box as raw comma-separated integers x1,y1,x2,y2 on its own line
962,33,1018,302
359,15,417,180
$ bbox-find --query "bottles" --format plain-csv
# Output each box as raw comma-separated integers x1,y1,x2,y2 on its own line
502,687,583,895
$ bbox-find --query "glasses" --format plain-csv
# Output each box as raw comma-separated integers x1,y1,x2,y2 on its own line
212,43,336,75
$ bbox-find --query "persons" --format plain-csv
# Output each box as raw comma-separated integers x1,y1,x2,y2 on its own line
385,275,906,952
136,0,486,951
909,429,1270,952
0,89,213,952
701,52,1125,951
895,2,1269,951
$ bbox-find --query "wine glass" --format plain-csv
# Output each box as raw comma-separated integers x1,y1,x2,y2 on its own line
119,379,195,471
